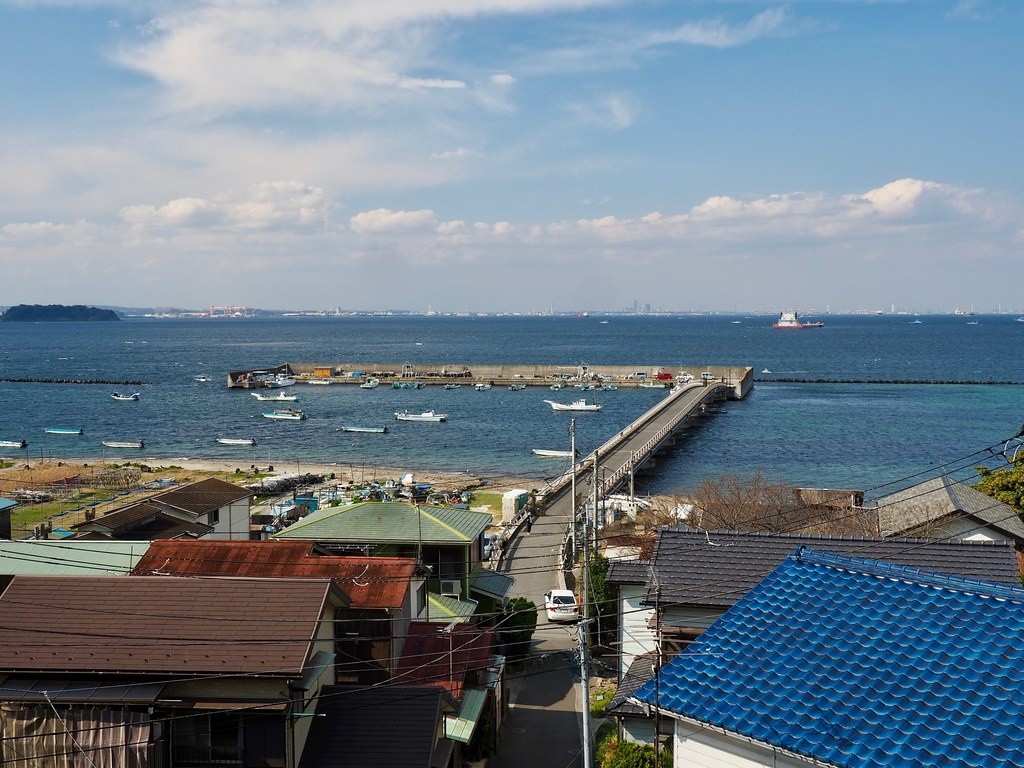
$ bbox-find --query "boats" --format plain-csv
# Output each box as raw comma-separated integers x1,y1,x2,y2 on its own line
263,409,309,420
309,379,334,386
391,381,399,389
195,376,211,382
597,385,604,390
45,427,83,434
359,377,380,389
544,398,602,412
532,448,577,457
580,383,591,390
550,384,560,390
102,440,144,448
252,392,297,402
266,362,296,387
607,384,617,391
508,384,525,390
395,411,448,421
341,426,388,433
415,381,427,388
475,383,492,391
0,439,25,448
443,384,461,389
640,382,665,388
112,392,140,400
401,382,413,388
216,438,255,445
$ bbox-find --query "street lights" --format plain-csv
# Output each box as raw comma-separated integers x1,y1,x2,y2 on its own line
643,703,659,768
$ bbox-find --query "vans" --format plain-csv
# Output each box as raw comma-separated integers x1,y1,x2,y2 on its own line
627,372,646,380
275,373,292,379
700,372,716,380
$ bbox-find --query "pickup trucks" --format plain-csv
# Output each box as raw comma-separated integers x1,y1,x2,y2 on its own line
676,373,695,380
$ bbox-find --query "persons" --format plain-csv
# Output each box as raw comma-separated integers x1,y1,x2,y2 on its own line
525,511,533,536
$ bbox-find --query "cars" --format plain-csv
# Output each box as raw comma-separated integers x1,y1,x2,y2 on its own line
655,373,672,380
543,589,580,623
484,533,497,560
